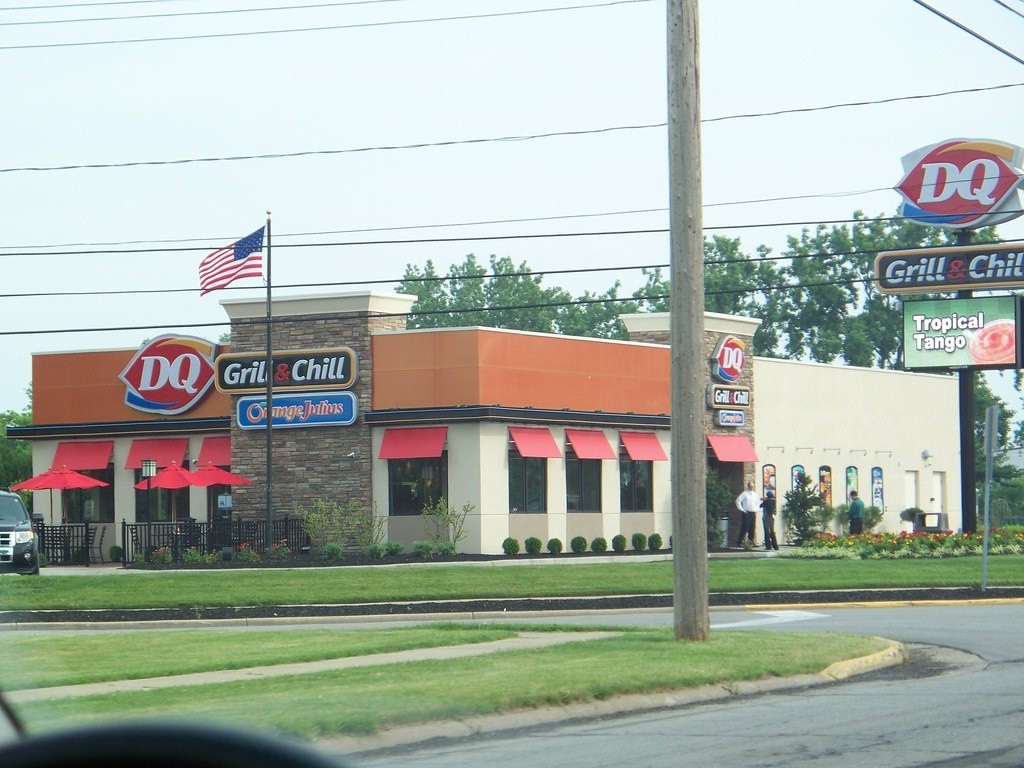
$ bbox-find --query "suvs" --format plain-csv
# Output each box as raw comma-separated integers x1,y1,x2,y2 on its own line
0,487,44,578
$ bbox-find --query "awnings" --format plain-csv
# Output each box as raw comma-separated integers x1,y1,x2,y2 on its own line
197,436,231,466
52,441,112,469
621,432,667,461
566,430,616,460
378,426,447,459
125,438,188,468
708,435,759,461
510,427,563,459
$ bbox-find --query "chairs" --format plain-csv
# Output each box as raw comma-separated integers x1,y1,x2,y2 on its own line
75,524,106,564
130,527,147,555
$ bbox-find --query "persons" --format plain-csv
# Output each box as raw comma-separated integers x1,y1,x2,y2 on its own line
736,483,761,547
760,491,779,550
849,491,865,534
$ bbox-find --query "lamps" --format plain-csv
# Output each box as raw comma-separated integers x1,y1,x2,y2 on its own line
301,546,311,554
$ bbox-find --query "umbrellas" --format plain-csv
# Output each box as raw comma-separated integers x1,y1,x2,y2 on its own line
134,464,192,523
11,469,109,531
191,465,252,522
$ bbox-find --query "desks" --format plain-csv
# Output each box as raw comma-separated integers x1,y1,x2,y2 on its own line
151,534,191,562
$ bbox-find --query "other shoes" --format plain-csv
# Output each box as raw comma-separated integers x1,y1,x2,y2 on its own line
760,548,771,552
772,547,778,551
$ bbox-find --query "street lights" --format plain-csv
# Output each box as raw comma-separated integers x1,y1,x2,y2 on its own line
140,459,158,562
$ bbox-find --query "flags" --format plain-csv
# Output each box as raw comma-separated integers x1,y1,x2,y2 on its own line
199,226,263,296
